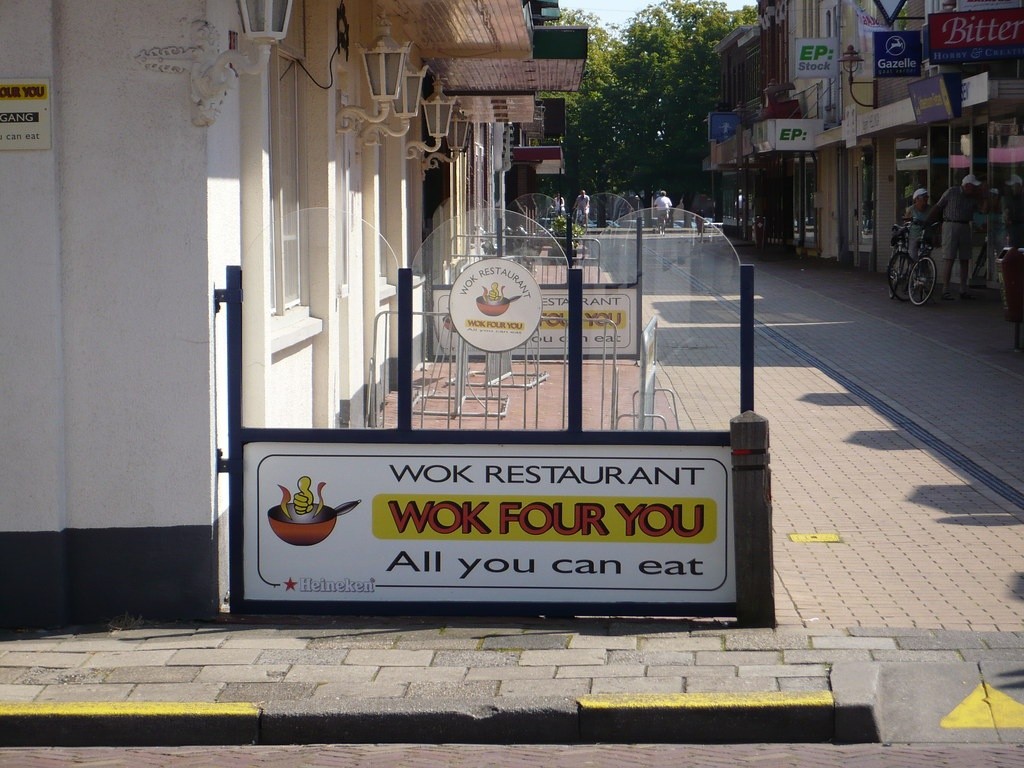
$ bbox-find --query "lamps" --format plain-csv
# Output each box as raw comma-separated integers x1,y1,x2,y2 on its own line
334,6,415,137
837,45,878,109
355,63,429,161
189,0,293,126
420,101,473,183
406,75,459,160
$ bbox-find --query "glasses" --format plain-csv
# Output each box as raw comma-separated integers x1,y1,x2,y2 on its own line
917,195,929,199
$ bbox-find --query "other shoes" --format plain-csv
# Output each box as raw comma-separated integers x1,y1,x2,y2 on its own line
941,292,954,301
904,282,912,294
959,290,971,300
920,290,927,305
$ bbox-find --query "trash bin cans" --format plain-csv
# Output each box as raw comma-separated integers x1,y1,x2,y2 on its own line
994,247,1024,323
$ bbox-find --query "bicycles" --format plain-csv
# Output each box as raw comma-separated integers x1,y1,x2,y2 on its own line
658,217,666,237
885,221,914,301
908,219,946,306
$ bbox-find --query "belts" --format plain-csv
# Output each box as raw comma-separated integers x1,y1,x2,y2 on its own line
944,220,969,224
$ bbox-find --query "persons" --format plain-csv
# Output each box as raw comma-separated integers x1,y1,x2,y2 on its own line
904,188,937,294
986,173,1024,283
920,173,989,302
551,193,566,216
654,190,673,225
572,189,590,227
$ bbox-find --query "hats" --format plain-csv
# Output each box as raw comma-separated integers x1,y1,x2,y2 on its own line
1005,174,1022,187
961,174,981,187
912,188,927,200
989,188,1000,195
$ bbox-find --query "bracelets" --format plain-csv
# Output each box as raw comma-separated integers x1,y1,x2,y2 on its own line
983,196,988,200
